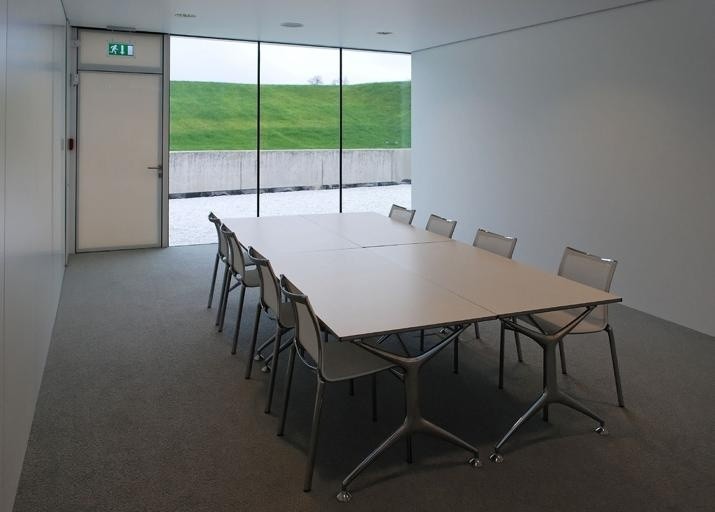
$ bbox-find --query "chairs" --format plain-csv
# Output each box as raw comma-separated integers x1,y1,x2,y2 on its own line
207,204,628,501
